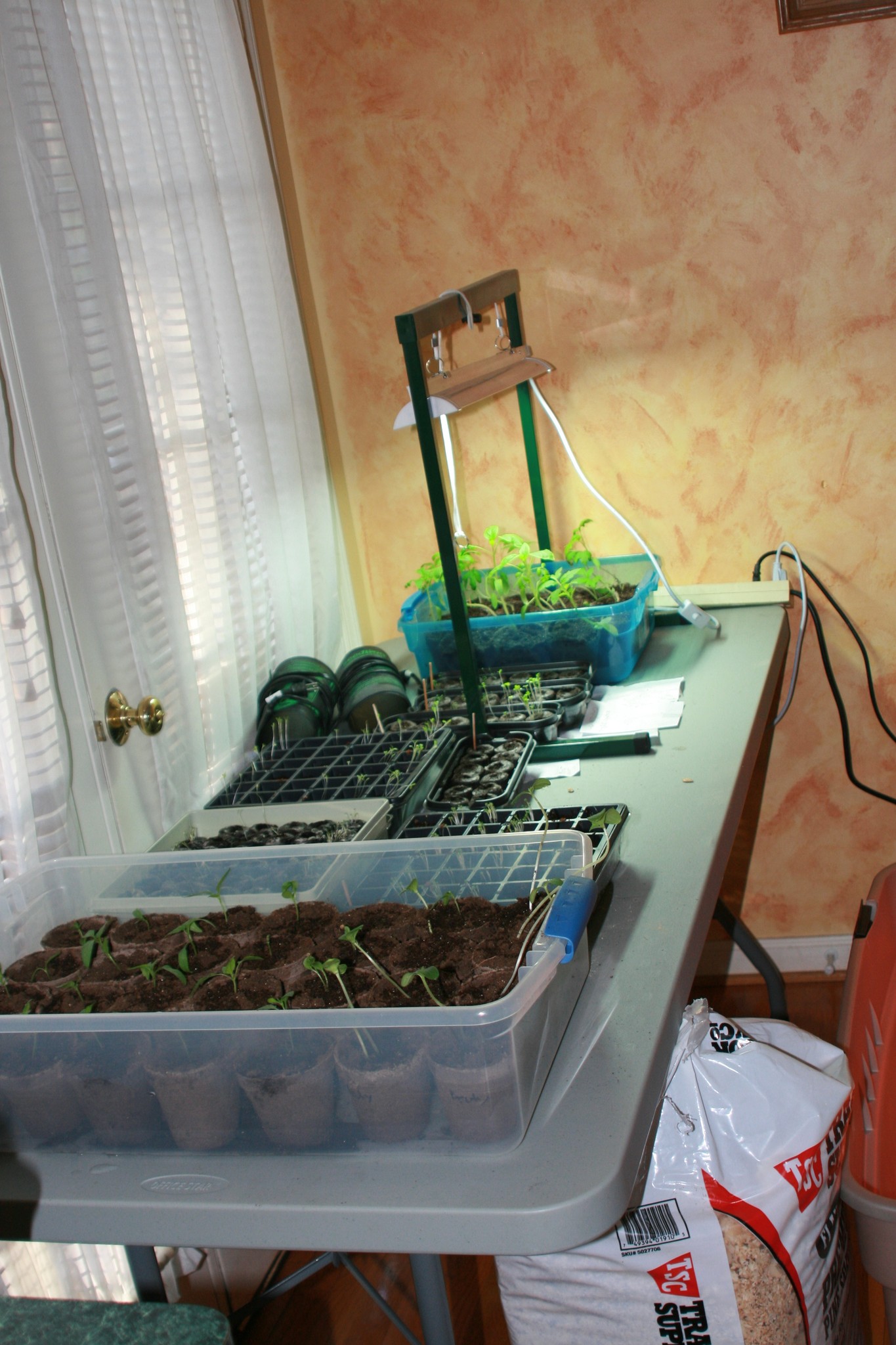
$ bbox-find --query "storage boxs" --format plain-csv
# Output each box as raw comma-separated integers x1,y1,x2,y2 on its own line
0,826,597,1151
398,551,665,686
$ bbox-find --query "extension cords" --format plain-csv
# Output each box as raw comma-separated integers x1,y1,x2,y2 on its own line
646,578,793,608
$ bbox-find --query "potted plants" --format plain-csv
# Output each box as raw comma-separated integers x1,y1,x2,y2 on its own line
0,517,653,1150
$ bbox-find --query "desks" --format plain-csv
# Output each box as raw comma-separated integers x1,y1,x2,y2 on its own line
0,600,793,1345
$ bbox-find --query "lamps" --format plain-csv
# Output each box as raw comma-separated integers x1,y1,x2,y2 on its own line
391,343,558,431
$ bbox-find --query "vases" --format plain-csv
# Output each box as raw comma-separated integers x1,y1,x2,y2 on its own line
461,752,489,768
480,771,510,790
472,782,503,796
484,760,515,779
335,902,418,946
450,771,480,790
469,744,495,763
443,783,475,801
490,751,521,767
